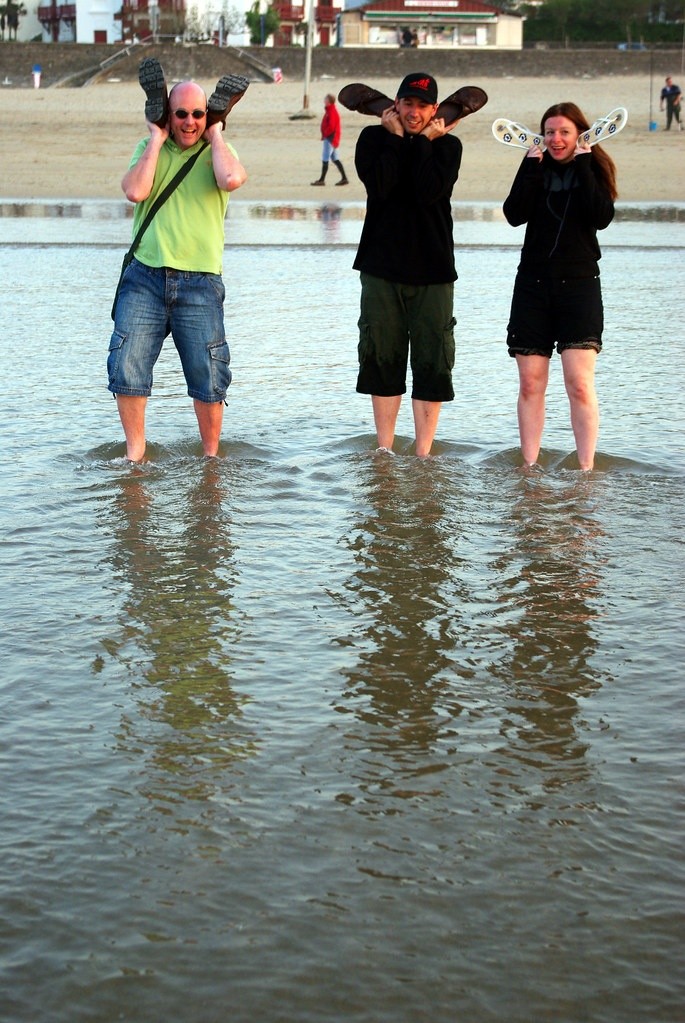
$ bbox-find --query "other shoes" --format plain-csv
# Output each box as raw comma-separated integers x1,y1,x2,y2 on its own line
311,179,325,186
336,179,348,186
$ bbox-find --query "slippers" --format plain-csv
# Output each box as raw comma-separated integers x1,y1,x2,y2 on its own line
576,106,628,149
338,83,400,117
492,119,548,153
432,87,488,127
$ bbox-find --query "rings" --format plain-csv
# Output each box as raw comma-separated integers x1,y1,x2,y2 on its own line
435,122,440,125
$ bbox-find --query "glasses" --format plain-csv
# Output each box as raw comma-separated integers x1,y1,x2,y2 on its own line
170,109,208,119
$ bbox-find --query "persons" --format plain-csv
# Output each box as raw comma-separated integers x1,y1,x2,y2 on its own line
403,27,418,48
310,94,348,186
352,74,462,457
107,81,247,463
503,102,617,471
660,77,682,130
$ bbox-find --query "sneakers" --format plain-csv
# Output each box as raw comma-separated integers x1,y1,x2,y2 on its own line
206,74,250,131
138,57,169,129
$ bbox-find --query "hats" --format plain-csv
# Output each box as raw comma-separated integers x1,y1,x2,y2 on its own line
397,71,437,105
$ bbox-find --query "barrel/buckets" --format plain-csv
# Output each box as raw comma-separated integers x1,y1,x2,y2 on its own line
649,121,656,131
